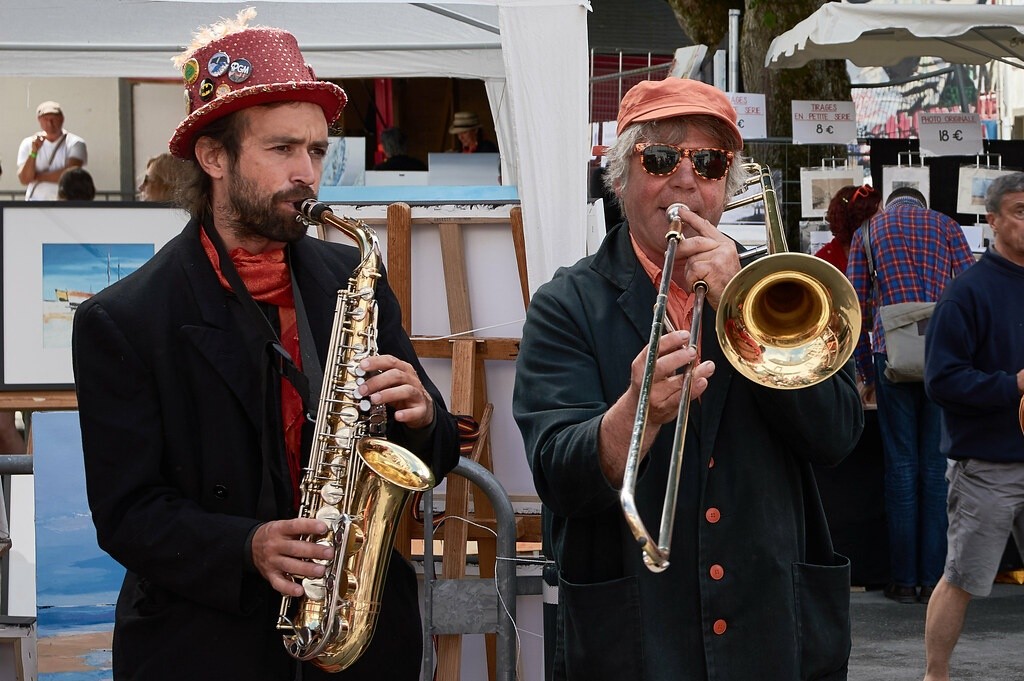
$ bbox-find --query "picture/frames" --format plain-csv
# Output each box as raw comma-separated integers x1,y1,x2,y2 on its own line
0,202,193,391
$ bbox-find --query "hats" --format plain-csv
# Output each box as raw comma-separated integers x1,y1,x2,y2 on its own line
886,187,926,211
449,112,482,135
36,101,61,118
615,77,744,150
169,8,347,159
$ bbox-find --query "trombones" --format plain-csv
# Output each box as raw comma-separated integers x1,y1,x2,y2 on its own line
614,198,866,575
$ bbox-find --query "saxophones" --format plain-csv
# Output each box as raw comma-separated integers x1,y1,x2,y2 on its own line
269,194,440,676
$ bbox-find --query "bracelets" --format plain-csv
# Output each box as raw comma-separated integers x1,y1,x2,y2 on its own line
30,152,37,158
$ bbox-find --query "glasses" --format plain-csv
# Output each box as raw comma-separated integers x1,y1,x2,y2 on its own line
633,142,734,182
850,184,874,203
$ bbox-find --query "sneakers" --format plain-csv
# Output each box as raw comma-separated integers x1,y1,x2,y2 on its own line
918,588,933,603
883,581,916,604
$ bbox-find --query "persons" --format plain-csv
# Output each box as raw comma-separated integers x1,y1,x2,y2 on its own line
925,170,1024,680
512,78,863,681
847,188,977,604
373,126,429,171
448,112,498,154
58,167,96,201
17,101,88,199
72,6,461,681
136,153,200,202
815,184,886,592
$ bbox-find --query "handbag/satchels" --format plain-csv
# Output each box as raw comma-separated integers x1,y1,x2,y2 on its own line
879,301,938,382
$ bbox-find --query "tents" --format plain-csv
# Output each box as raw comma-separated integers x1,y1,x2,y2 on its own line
763,0,1024,74
